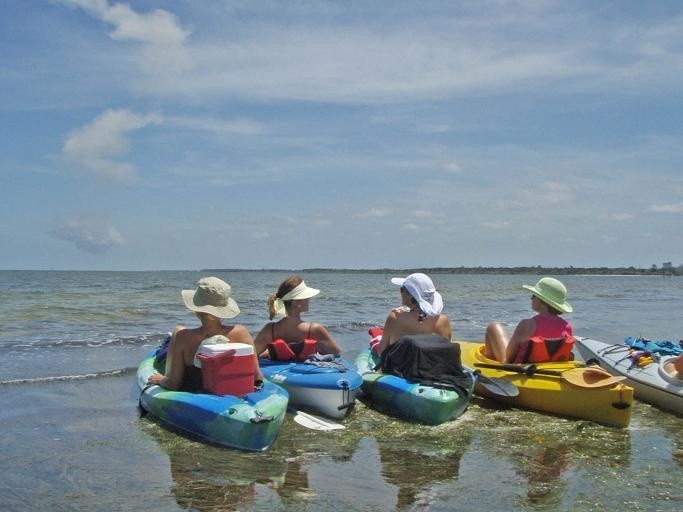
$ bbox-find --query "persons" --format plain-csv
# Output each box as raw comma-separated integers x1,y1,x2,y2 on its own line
252,277,341,361
484,276,575,363
367,273,452,358
145,276,263,391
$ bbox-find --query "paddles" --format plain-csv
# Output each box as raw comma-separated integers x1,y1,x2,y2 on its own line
474,362,627,390
286,406,345,431
463,364,520,397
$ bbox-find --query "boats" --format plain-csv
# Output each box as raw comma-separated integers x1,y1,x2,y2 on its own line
355,345,479,427
136,331,290,452
254,354,365,422
572,335,683,417
451,338,636,429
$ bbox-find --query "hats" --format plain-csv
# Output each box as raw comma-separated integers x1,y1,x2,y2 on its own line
181,276,240,319
273,279,320,315
391,273,443,318
522,277,573,313
